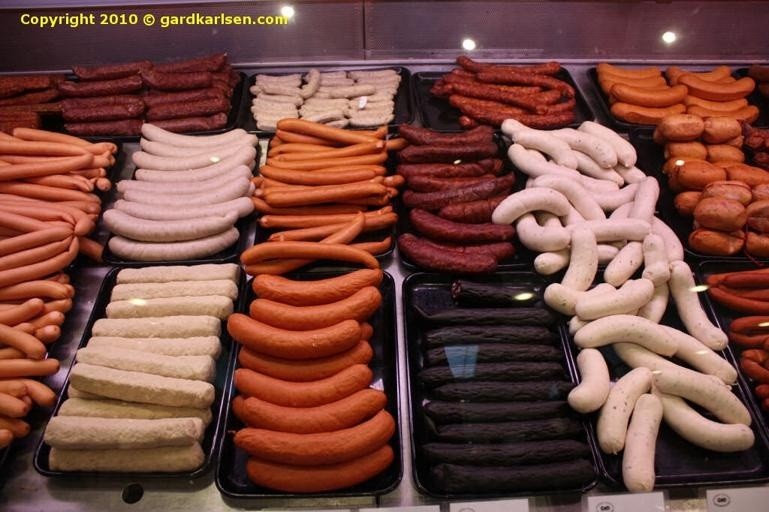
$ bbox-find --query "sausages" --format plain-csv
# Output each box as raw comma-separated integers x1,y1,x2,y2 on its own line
1,52,768,497
